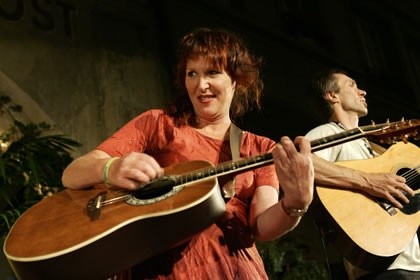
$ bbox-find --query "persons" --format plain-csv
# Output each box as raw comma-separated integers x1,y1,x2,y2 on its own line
301,68,420,280
59,24,316,279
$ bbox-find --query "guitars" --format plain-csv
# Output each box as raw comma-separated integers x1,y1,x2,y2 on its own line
2,117,420,280
310,140,419,272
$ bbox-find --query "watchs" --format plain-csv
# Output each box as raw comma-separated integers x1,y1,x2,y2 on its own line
280,199,309,216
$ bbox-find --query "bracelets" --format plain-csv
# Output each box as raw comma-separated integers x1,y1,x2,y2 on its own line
103,157,121,188
